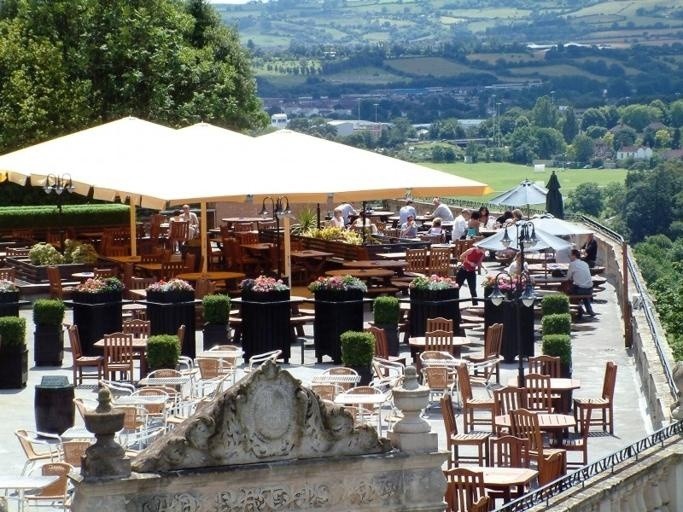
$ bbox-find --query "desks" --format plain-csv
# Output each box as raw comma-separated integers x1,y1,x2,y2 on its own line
175,271,245,289
60,425,97,441
324,267,394,279
311,375,362,389
106,255,152,265
532,274,607,285
209,228,264,235
352,209,394,217
333,393,386,438
507,376,581,414
199,351,245,367
375,251,446,259
289,248,336,286
405,335,472,347
421,220,459,230
388,215,433,222
1,474,60,512
240,242,277,276
494,413,576,449
478,226,499,233
525,253,558,261
114,394,168,412
71,271,94,279
128,289,150,299
135,263,191,279
343,259,408,269
426,358,470,369
93,335,148,381
137,377,193,387
221,216,273,223
528,263,571,271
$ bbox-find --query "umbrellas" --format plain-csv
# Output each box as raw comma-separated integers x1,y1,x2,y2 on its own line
0,115,493,287
530,218,595,236
472,221,569,256
489,180,568,220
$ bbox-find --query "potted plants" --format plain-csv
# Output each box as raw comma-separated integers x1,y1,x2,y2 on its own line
203,294,231,351
0,316,28,390
147,334,180,380
540,333,573,413
340,330,376,387
33,298,65,367
373,296,400,356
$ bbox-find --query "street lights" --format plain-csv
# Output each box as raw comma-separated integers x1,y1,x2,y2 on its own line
500,222,540,272
488,273,536,389
42,173,76,254
259,196,293,281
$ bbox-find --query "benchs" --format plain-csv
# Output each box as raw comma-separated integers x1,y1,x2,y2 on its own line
566,293,596,322
533,287,606,293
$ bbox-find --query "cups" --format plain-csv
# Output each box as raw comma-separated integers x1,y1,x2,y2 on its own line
542,261,546,266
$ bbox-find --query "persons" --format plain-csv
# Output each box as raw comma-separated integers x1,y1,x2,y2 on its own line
509,253,529,275
427,218,445,237
581,233,598,268
399,215,418,238
335,202,357,224
451,209,470,240
169,209,185,236
478,206,490,229
399,199,417,228
510,209,526,223
180,204,199,239
456,246,485,305
330,208,344,227
567,249,596,317
466,211,481,240
352,211,371,226
424,198,454,221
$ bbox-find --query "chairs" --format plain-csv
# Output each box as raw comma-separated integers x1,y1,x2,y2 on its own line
14,428,63,476
528,355,561,414
93,267,112,279
311,384,345,406
455,238,475,261
23,462,74,512
426,317,453,338
129,277,144,289
454,362,502,434
141,255,160,264
322,367,358,387
178,324,185,346
0,266,15,287
224,238,257,273
487,436,531,507
194,358,232,398
241,232,259,253
234,222,255,234
68,325,105,388
442,467,490,512
47,264,73,300
428,248,450,279
493,385,546,450
130,389,168,424
61,441,90,468
161,261,184,280
279,240,308,284
420,350,454,367
243,349,278,376
440,393,494,469
103,332,133,387
425,330,454,357
122,319,150,379
572,361,617,435
547,399,593,468
258,221,277,230
132,265,145,279
405,246,428,275
172,220,189,249
468,323,503,384
113,406,145,451
146,369,184,401
517,372,555,414
345,386,382,422
507,408,567,487
446,466,540,511
425,366,455,406
364,327,406,389
98,379,135,406
